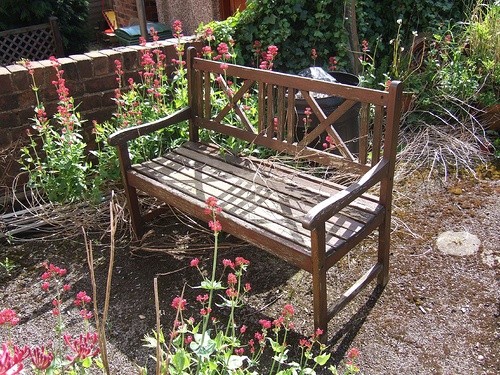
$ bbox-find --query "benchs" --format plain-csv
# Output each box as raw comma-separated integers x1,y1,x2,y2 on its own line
107,47,403,346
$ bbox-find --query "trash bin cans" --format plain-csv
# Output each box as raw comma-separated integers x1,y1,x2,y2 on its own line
282,67,360,171
115,21,173,46
